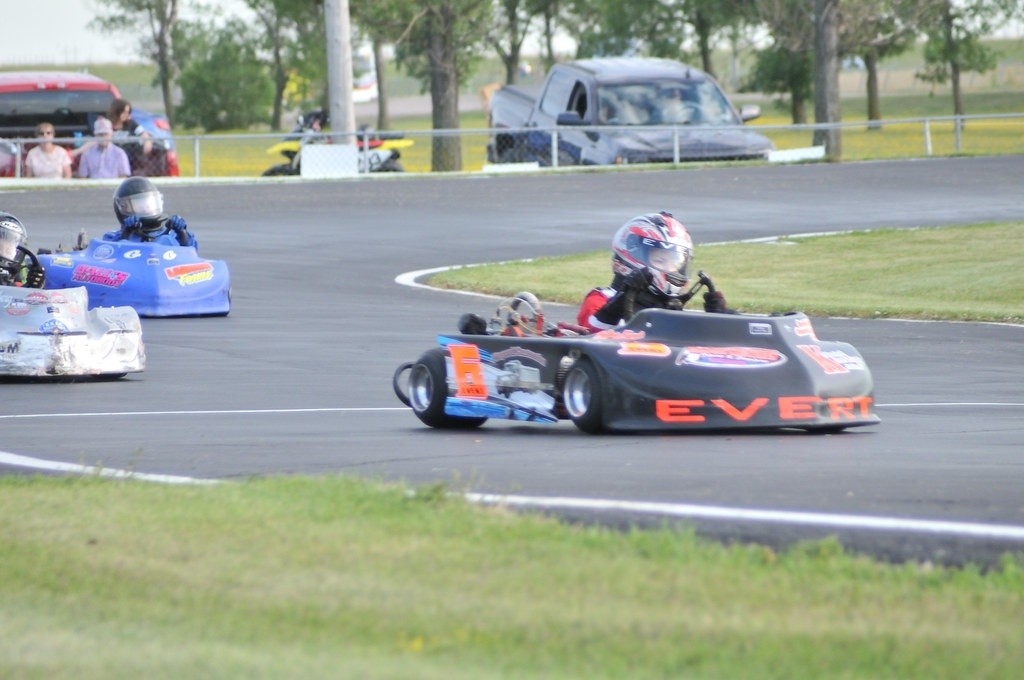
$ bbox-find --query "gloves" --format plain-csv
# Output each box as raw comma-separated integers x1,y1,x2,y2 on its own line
165,215,192,246
702,291,742,317
27,266,45,288
594,267,653,326
121,215,143,237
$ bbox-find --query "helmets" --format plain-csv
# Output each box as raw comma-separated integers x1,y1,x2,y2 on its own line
114,176,164,231
611,211,693,298
0,210,27,272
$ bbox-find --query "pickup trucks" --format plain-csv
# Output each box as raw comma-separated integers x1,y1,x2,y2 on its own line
486,56,775,167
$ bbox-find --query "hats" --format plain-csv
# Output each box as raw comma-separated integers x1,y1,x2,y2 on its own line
94,119,114,135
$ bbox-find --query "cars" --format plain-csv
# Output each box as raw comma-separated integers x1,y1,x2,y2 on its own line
128,102,173,154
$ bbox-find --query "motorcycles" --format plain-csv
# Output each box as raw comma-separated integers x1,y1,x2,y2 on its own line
258,105,416,175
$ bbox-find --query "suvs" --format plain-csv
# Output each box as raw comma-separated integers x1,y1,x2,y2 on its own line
0,70,180,178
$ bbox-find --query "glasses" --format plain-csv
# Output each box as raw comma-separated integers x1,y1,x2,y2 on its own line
39,132,52,135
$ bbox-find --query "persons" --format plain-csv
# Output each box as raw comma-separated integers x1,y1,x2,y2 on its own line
578,211,740,334
103,177,197,249
0,212,46,289
25,100,151,179
